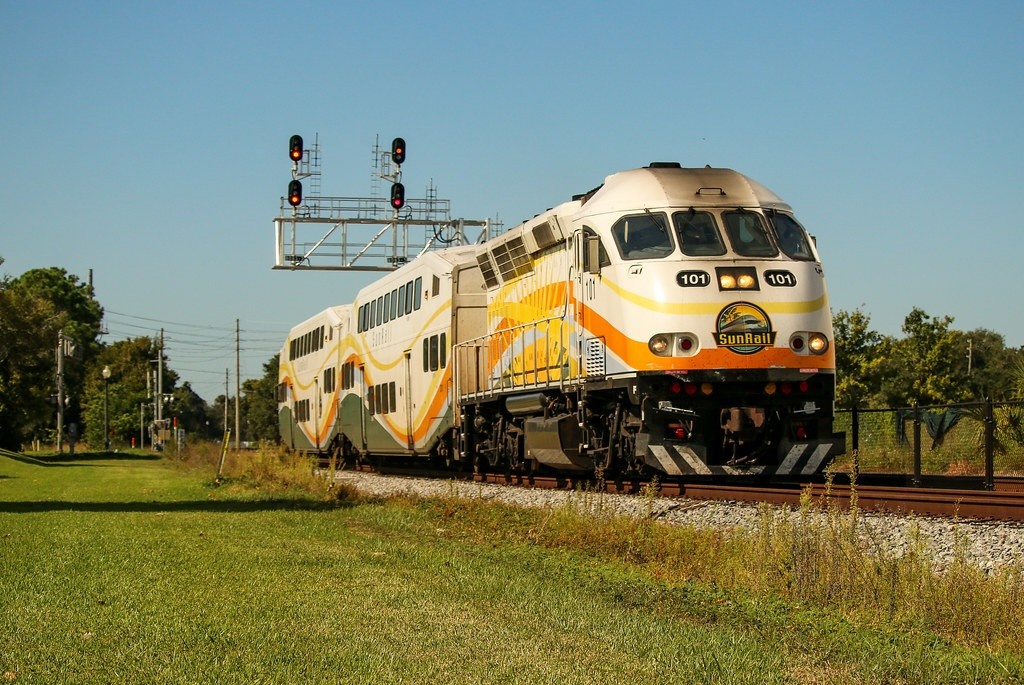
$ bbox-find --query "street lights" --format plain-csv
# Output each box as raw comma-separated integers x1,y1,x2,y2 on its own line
158,393,174,446
206,420,209,438
102,366,112,449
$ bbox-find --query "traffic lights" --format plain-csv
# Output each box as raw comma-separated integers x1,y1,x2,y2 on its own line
391,183,404,209
288,180,302,206
289,135,304,161
392,138,406,164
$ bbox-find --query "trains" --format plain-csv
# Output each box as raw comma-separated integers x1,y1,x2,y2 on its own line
272,161,847,479
